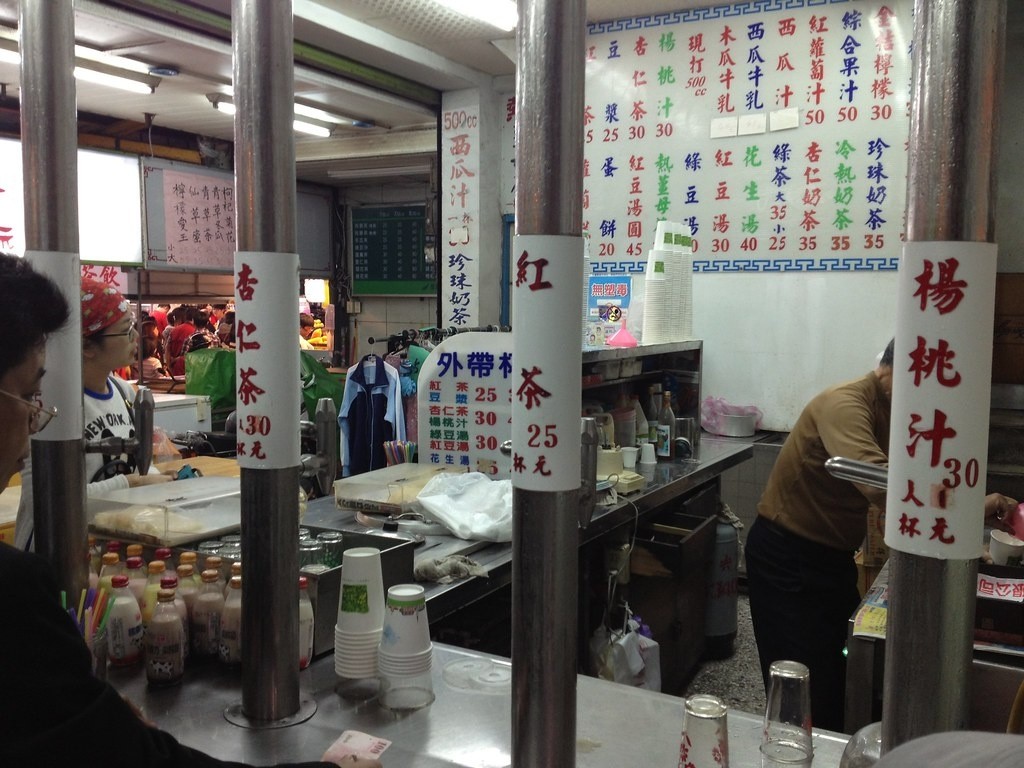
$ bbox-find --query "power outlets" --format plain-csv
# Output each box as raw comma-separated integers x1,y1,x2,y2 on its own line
604,542,629,584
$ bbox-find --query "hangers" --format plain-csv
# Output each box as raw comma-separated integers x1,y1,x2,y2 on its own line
361,326,509,376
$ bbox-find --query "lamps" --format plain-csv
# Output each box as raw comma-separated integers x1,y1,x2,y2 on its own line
206,92,336,137
0,38,162,94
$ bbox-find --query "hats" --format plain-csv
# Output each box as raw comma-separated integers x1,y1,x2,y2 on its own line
79,275,129,334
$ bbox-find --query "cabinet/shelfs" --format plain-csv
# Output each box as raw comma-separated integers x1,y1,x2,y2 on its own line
582,340,705,446
630,478,720,695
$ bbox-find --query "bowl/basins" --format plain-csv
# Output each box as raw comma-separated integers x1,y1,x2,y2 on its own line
719,414,755,437
989,530,1024,566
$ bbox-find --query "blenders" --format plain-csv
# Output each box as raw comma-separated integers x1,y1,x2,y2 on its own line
597,441,644,495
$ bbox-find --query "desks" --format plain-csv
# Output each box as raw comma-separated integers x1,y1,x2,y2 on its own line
103,440,853,767
132,367,186,393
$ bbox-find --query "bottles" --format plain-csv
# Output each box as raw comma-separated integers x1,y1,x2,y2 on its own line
88,535,198,665
610,406,637,447
205,556,224,593
225,562,242,601
219,576,242,664
334,546,434,709
657,391,676,460
145,588,185,686
191,570,223,655
645,386,659,450
300,577,315,668
617,392,649,447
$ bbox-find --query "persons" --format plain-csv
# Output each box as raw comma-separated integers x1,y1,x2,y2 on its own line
744,336,1018,733
299,313,315,351
0,253,343,768
132,303,235,383
79,277,174,495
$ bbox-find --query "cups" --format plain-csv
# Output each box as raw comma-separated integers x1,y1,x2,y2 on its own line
299,539,324,567
676,695,728,768
318,532,344,566
582,229,590,347
757,660,814,768
641,221,692,344
299,528,312,542
300,563,330,574
199,534,242,558
621,446,638,469
1009,503,1024,542
640,444,657,465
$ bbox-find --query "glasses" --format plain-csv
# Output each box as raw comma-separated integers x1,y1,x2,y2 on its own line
0,390,58,433
301,326,314,333
86,311,138,343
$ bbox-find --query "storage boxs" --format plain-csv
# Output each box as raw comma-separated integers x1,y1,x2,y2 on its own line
88,476,416,657
